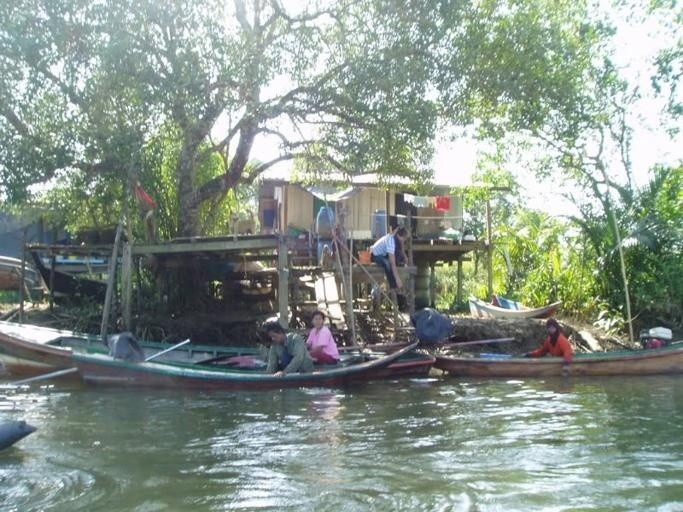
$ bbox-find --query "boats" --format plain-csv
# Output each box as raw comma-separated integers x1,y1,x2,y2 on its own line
435,346,683,377
1,314,421,392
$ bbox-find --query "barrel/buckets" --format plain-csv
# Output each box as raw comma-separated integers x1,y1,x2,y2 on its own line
371,209,385,239
317,207,334,238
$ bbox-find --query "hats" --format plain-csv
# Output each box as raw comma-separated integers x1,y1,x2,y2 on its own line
546,319,557,328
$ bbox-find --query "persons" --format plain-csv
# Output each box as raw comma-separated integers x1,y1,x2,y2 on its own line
370,227,411,289
520,318,572,364
266,311,339,377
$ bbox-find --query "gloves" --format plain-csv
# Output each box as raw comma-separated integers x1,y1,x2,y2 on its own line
560,362,571,376
